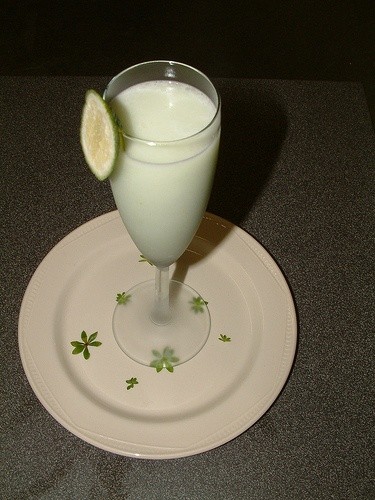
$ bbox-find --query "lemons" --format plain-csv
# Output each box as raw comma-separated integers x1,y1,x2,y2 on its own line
78,89,119,182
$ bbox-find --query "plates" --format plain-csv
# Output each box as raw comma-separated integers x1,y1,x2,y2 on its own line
17,208,297,461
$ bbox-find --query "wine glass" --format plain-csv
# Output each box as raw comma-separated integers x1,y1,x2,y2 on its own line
101,60,221,367
